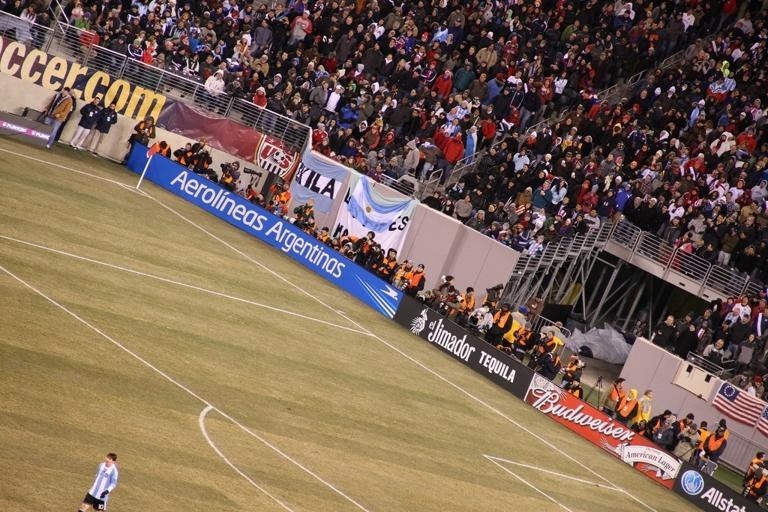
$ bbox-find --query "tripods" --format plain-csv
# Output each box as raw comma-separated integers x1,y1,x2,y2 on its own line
584,376,603,409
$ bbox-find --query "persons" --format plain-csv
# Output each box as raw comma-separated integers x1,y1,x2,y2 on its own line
602,398,768,512
1,0,767,118
316,120,768,400
78,452,118,511
42,118,315,237
